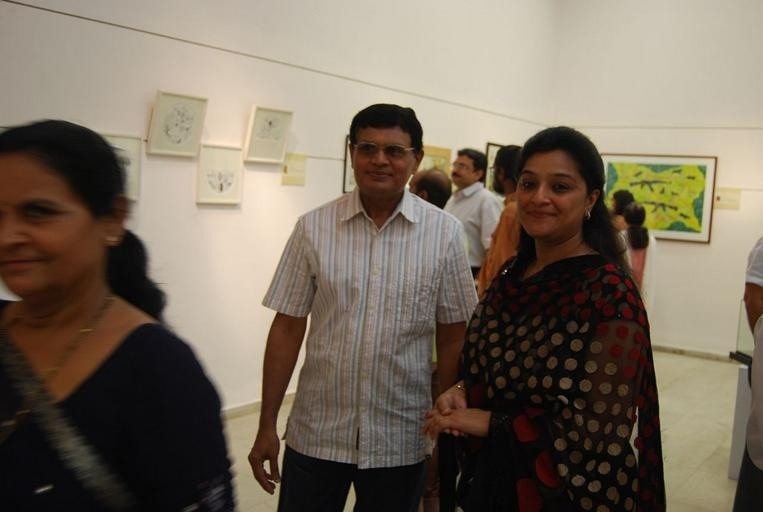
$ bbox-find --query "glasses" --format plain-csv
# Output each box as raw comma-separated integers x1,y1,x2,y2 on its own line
354,142,415,156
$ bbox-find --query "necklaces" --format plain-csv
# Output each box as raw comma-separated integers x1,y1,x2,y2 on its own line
1,290,116,444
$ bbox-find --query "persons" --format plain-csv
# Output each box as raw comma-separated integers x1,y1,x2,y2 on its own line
0,119,233,512
246,106,666,512
732,235,763,512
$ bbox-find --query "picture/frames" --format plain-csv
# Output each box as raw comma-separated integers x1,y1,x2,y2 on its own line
244,103,294,165
484,142,502,195
342,134,357,193
101,133,142,203
600,152,718,244
145,89,209,159
194,142,244,207
417,145,452,175
